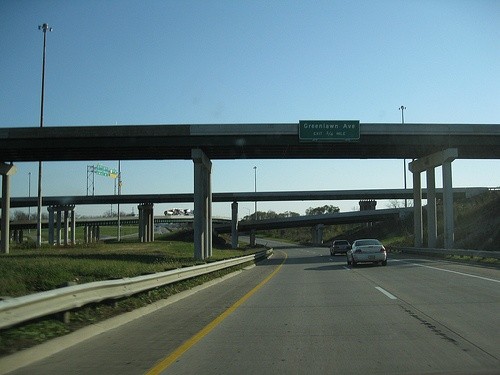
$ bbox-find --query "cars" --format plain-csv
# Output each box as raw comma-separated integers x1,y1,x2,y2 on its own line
329,240,352,256
345,238,387,266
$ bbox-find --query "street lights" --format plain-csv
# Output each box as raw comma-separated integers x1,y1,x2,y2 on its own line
252,166,257,220
399,105,408,208
28,172,32,238
36,23,53,249
